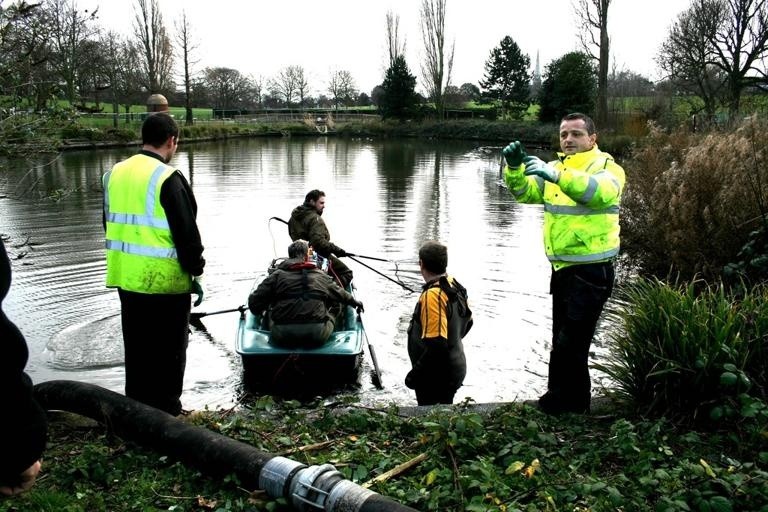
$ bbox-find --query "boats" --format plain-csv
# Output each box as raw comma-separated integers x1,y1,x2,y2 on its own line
235,258,364,373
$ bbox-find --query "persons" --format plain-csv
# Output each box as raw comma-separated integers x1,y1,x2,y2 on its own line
247,238,364,348
502,113,628,417
99,112,207,417
287,189,352,288
1,239,47,496
403,240,473,405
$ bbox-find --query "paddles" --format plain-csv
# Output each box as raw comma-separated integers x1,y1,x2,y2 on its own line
350,281,384,390
189,304,250,320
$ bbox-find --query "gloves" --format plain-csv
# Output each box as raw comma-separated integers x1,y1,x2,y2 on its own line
503,141,560,184
191,276,203,307
356,301,364,313
334,249,346,258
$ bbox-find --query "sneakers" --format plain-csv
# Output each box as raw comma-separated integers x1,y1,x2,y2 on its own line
523,392,591,414
